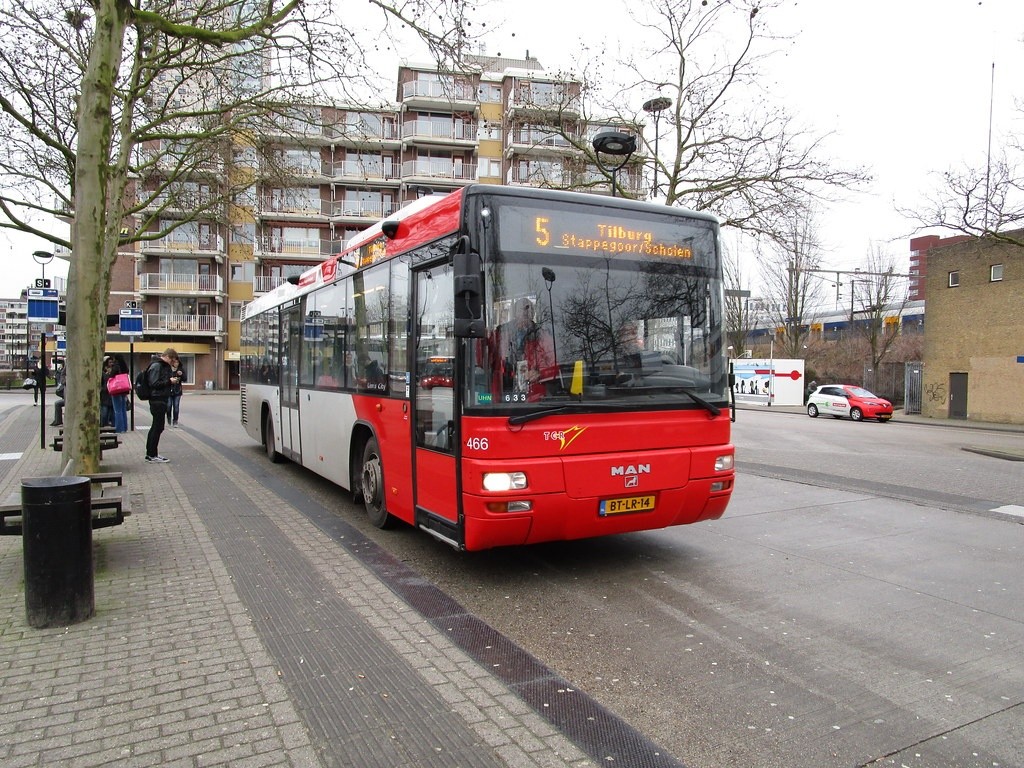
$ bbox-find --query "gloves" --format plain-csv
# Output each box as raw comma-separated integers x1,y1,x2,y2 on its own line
31,372,34,375
48,376,54,380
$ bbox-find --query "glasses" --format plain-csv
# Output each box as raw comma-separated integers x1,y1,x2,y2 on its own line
109,362,113,363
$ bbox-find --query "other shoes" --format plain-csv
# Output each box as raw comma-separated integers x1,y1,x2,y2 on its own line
145,455,150,462
166,424,170,427
49,421,63,427
173,423,178,427
149,455,170,463
33,402,37,406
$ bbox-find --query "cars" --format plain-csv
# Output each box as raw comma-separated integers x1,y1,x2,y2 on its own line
806,384,895,423
591,384,605,399
502,362,515,391
389,372,407,381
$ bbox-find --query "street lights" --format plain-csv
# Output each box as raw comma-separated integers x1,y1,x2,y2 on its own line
849,279,872,337
541,266,556,393
767,324,776,406
592,132,637,197
644,96,674,200
32,250,54,449
7,311,17,370
746,297,763,359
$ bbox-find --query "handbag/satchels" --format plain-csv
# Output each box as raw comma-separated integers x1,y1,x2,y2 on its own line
23,378,37,390
107,374,132,395
55,383,64,397
126,397,131,411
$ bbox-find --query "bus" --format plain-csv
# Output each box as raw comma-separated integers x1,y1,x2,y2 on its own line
238,183,739,561
421,355,456,387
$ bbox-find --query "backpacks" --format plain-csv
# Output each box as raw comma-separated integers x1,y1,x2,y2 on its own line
133,361,163,400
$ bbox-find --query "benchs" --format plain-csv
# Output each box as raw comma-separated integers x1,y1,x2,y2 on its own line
0,427,132,536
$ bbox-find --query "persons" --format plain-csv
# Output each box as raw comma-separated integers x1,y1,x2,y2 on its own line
145,349,187,463
312,354,384,388
477,299,557,403
241,358,279,385
99,354,129,434
50,365,66,426
32,357,53,406
619,315,646,356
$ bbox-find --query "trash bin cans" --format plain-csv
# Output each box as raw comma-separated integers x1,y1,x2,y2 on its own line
19,475,96,630
204,380,214,391
